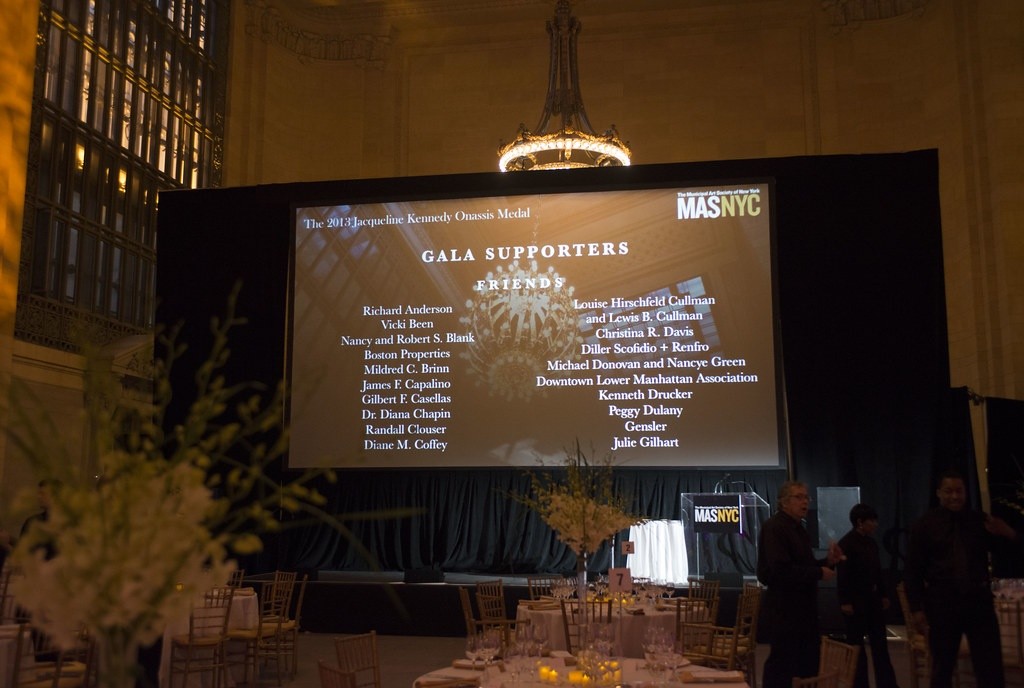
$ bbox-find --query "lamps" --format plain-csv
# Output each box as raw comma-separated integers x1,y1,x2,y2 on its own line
457,195,586,404
496,0,633,171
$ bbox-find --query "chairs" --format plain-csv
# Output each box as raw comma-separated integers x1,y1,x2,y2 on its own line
897,576,1024,688
818,636,859,688
317,657,357,688
0,567,308,688
791,665,840,688
411,572,763,688
334,629,381,688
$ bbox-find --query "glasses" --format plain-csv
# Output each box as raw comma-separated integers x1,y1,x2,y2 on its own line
789,493,809,500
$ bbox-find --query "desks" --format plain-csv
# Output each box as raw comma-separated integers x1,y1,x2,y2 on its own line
0,624,35,688
412,655,752,688
157,591,260,688
516,594,711,657
991,586,1024,664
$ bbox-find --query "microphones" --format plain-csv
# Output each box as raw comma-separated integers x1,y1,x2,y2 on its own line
714,474,755,494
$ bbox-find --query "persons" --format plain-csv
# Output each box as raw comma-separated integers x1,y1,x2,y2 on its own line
756,481,833,688
13,480,75,661
834,505,900,688
904,467,1023,688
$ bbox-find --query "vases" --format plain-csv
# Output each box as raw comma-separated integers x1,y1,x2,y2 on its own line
576,550,588,652
94,625,138,688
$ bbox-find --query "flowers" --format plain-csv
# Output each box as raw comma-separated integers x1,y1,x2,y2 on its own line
514,435,667,569
1,302,431,676
